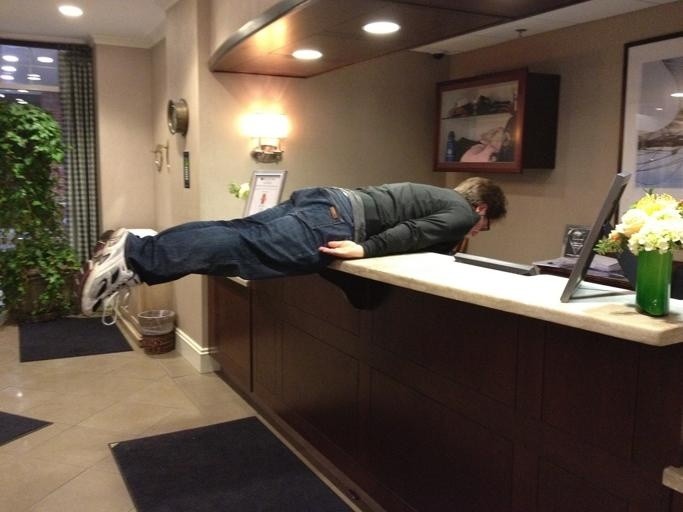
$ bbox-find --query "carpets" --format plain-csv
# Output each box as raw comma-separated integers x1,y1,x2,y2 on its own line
19,316,133,362
108,416,354,512
0,411,53,446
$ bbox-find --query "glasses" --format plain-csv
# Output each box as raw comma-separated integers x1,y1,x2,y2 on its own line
479,213,491,232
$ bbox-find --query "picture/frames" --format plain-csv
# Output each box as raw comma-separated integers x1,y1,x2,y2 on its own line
615,31,683,229
242,170,288,218
561,173,636,303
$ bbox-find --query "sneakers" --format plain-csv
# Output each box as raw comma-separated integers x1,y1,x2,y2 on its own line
76,227,141,327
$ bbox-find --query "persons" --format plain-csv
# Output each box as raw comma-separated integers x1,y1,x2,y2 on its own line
80,175,510,328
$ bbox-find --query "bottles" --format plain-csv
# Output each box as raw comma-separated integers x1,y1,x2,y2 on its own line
444,130,457,160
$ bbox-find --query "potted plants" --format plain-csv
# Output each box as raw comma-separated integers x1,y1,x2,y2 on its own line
0,94,82,324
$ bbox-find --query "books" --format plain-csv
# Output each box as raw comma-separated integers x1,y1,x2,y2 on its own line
587,253,623,272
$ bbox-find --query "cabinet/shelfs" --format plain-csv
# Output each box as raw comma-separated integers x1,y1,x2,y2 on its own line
433,66,560,175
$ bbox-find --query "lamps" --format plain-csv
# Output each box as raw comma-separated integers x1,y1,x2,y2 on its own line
249,112,284,163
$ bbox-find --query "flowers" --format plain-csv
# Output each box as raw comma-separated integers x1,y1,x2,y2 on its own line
593,188,683,256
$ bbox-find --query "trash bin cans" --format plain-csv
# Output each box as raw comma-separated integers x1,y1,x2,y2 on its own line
135,309,176,356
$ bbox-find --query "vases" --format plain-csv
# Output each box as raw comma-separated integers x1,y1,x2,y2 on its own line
636,251,673,316
